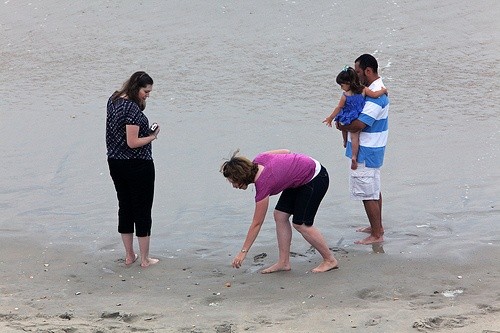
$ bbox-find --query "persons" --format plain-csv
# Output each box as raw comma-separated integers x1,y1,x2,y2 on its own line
336,54,389,244
106,71,160,267
322,65,388,169
219,148,338,274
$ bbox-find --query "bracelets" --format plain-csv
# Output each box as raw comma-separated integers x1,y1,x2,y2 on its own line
241,249,248,253
